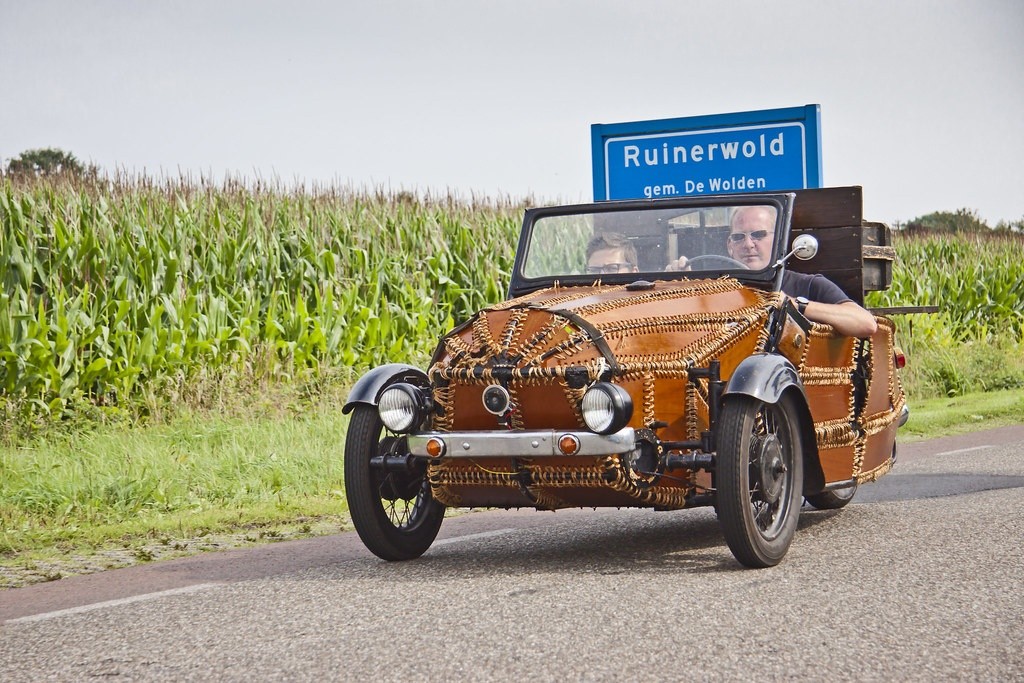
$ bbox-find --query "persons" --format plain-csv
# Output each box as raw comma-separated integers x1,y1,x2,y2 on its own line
585,233,639,274
665,205,877,337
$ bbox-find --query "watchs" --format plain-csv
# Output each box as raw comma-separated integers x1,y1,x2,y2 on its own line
795,296,809,315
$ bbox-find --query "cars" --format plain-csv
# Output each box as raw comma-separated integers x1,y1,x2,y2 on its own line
341,185,941,569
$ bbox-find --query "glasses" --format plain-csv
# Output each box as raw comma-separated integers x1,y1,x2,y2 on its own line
584,263,634,274
730,230,775,243
